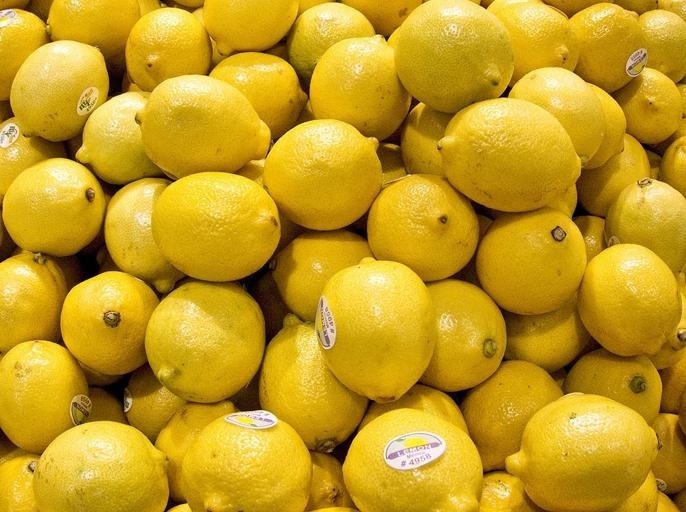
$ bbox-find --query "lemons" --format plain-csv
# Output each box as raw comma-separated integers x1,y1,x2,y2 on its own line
0,0,686,512
